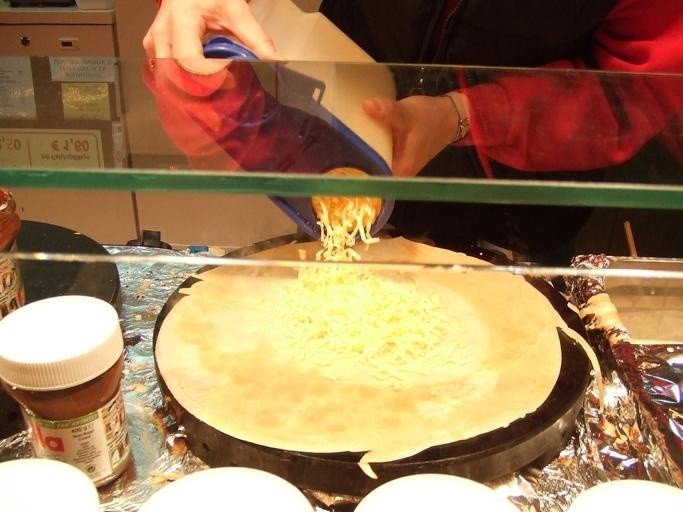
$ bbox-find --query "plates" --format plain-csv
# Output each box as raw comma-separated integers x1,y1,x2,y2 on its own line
16,220,120,314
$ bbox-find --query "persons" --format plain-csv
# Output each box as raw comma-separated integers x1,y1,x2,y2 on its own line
141,0,682,265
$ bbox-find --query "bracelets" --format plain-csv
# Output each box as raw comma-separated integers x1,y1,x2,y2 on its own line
447,88,469,142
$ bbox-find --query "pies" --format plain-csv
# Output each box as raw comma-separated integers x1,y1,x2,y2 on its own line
154,238,562,480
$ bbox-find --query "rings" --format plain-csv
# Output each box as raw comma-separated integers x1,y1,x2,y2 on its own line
146,57,156,72
